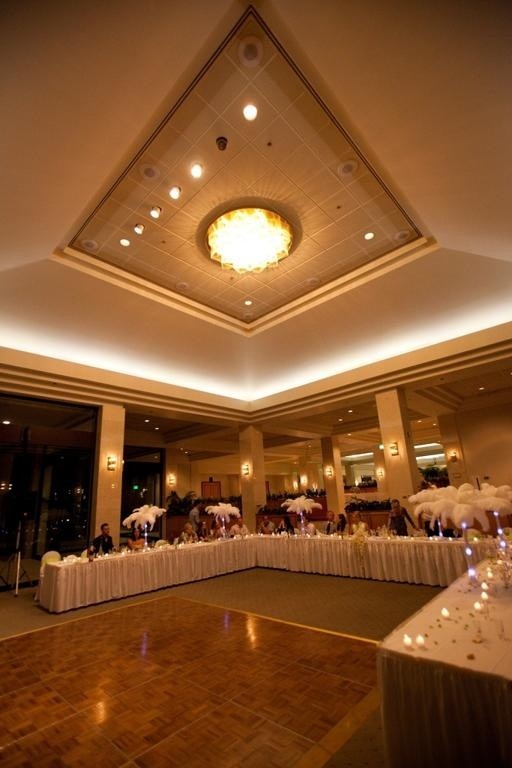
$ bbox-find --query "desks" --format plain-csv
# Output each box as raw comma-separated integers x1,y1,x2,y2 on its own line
37,534,257,613
374,543,511,767
258,534,508,588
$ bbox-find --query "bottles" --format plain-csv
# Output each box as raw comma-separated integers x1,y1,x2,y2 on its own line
88,541,94,562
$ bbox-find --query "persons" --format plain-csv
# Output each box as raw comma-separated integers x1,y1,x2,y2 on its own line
297,515,316,535
334,512,350,536
321,511,337,535
176,500,248,546
93,523,115,556
275,515,295,534
350,511,370,537
387,477,462,537
259,514,275,534
127,527,145,550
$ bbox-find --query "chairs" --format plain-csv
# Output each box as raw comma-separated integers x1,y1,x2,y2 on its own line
34,551,63,603
